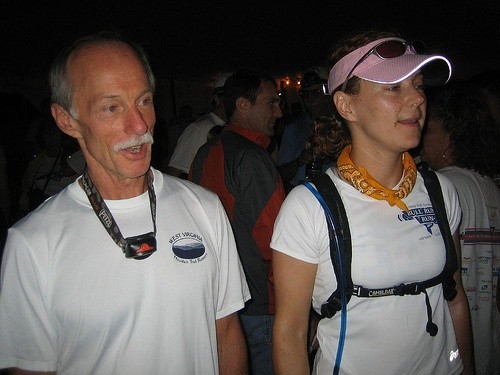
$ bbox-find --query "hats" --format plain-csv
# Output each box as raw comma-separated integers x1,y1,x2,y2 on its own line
325,36,453,87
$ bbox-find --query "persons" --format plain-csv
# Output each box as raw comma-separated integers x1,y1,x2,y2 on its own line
17,64,500,375
0,32,252,375
269,28,476,375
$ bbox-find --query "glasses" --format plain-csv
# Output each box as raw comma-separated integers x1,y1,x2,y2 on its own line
341,39,427,92
